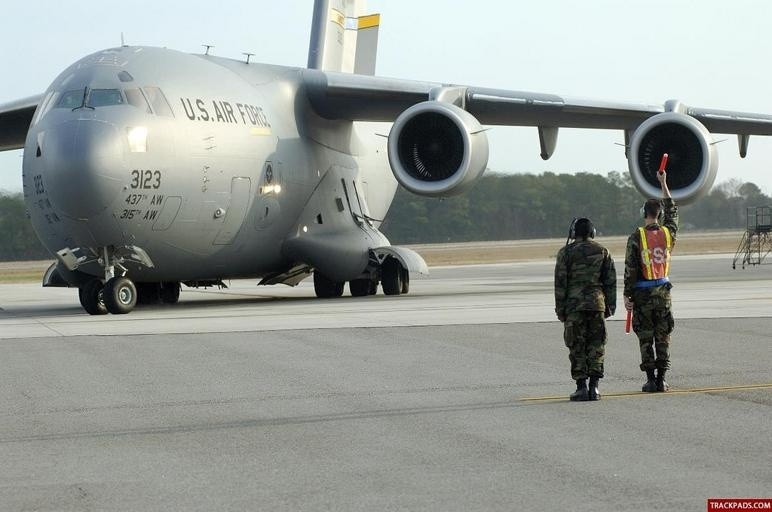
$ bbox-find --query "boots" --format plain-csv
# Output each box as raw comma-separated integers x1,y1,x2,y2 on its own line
570,376,601,400
642,367,669,392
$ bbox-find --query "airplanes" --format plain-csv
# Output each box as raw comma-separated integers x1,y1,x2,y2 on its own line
0,0,772,317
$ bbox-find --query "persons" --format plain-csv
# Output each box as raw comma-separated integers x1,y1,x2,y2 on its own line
554,216,617,401
623,169,679,392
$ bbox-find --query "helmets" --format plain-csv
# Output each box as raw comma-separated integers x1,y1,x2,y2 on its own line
575,219,593,234
